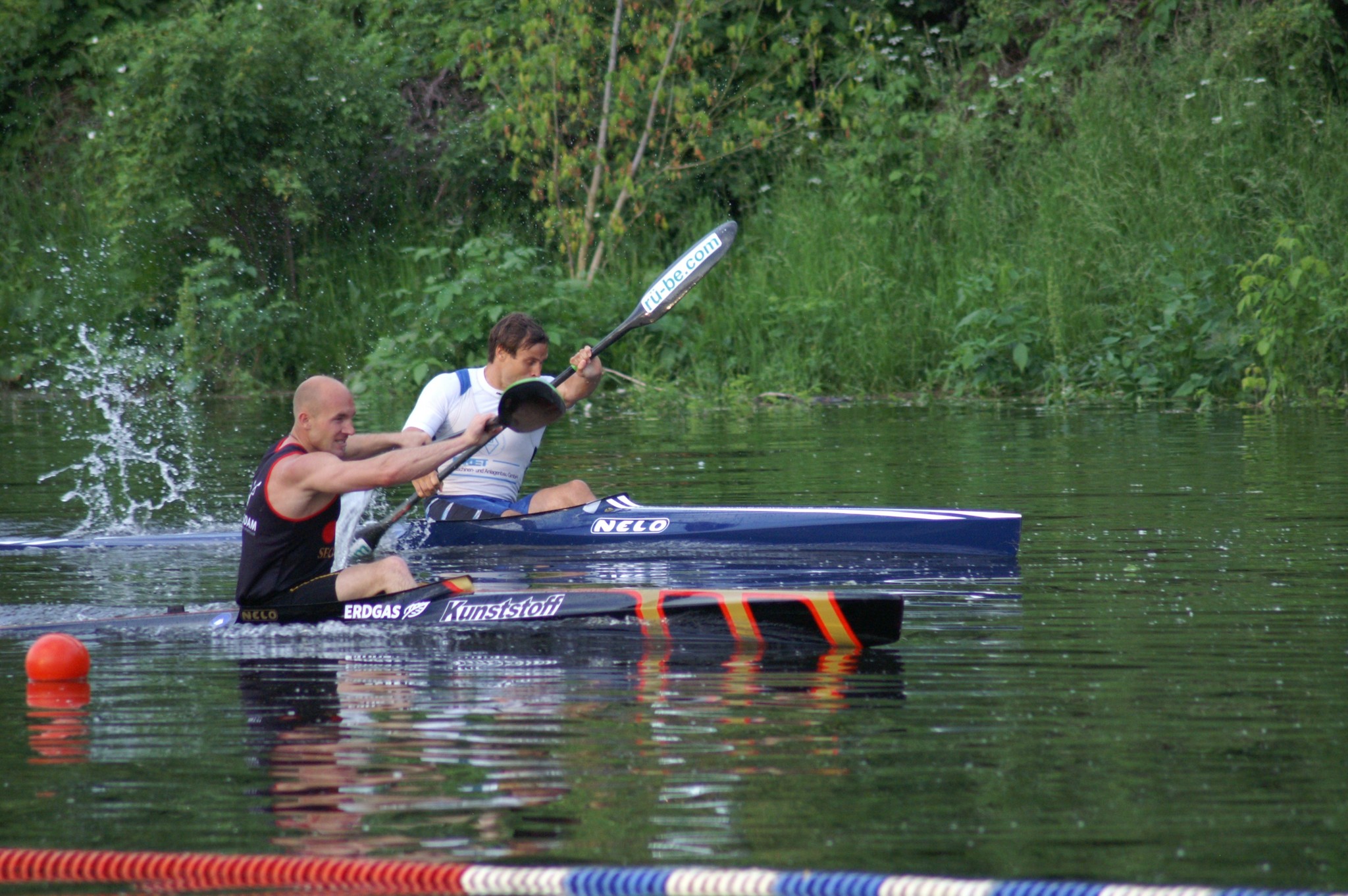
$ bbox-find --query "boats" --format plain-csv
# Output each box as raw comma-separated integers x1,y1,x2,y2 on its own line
0,491,1024,552
1,574,905,637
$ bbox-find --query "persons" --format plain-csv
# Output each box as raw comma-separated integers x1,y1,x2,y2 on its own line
400,312,604,523
234,374,502,611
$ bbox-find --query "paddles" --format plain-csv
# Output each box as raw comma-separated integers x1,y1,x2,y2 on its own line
429,377,568,446
347,218,740,561
